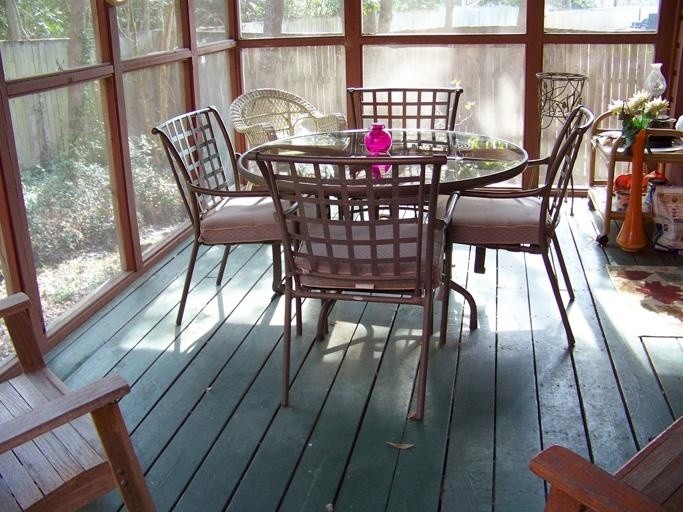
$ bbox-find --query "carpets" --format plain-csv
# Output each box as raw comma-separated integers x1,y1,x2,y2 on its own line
607,264,682,337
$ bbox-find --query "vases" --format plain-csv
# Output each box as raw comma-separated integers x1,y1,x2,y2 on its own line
616,129,648,254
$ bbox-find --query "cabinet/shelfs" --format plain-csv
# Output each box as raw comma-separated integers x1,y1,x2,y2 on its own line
588,110,683,246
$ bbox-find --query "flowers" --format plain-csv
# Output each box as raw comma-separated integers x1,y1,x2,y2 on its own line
607,88,671,149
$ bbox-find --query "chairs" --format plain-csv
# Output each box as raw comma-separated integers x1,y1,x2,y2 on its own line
529,414,682,512
151,105,319,335
347,87,464,130
229,88,348,148
428,104,594,349
247,151,460,420
0,292,155,512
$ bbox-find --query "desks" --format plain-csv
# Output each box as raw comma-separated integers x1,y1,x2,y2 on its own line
238,129,530,347
